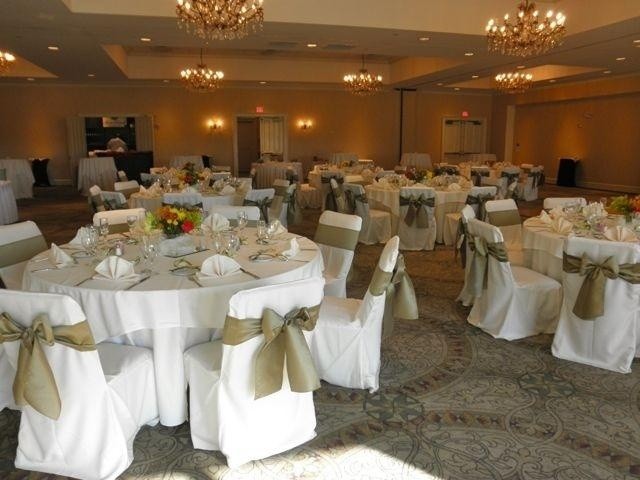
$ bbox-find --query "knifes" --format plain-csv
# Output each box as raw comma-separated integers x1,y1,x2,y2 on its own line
240,268,261,280
122,275,151,293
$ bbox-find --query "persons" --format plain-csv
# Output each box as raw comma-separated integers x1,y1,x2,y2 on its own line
105,134,129,151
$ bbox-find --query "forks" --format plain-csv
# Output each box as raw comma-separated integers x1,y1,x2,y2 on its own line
188,277,203,288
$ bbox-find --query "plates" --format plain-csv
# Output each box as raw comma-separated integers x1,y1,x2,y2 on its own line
71,230,279,278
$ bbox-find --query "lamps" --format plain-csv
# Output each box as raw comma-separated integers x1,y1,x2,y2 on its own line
485,0,568,59
180,47,224,91
494,72,534,95
174,0,265,42
343,57,383,97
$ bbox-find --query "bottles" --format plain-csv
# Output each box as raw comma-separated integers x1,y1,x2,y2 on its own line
113,240,124,256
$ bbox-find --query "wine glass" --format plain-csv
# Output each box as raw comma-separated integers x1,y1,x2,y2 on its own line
157,175,238,193
563,196,608,228
79,210,267,275
